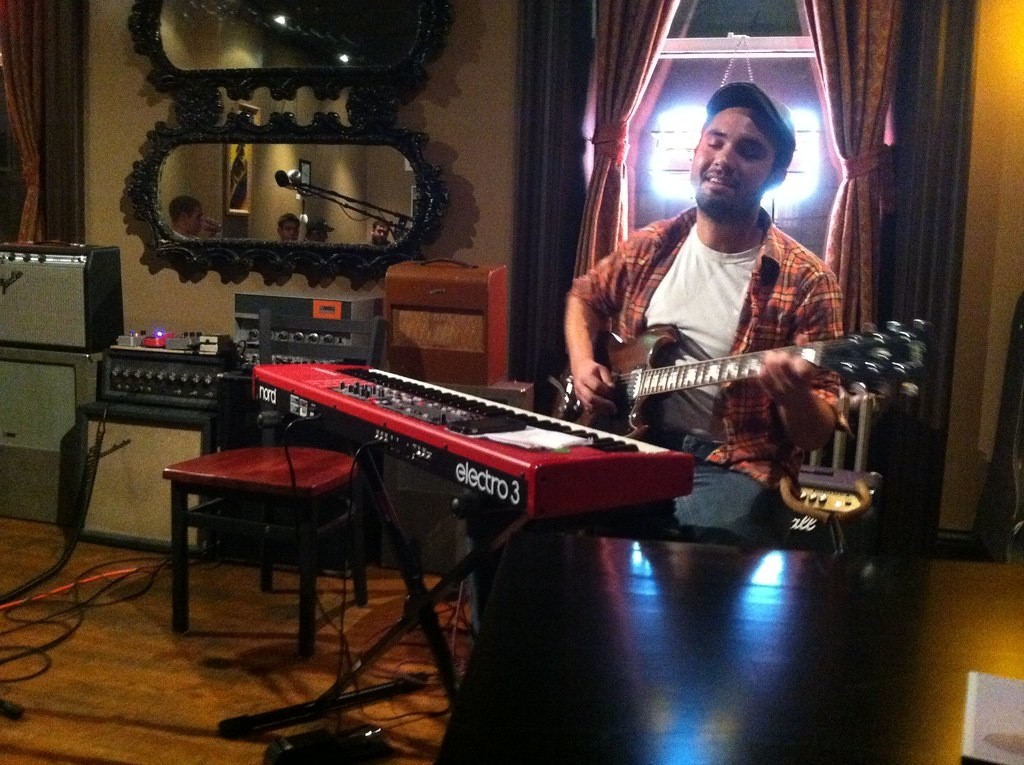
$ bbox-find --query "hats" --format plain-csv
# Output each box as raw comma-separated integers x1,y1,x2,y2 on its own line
304,216,336,231
277,211,301,227
706,81,797,155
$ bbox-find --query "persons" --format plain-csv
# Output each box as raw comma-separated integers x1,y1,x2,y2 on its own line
464,82,856,640
278,213,300,240
368,220,391,245
169,195,204,241
306,216,335,242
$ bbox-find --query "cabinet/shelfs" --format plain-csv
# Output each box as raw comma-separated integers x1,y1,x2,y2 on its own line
1,348,102,526
78,401,216,555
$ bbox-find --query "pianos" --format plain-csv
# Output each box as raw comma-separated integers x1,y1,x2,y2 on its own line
251,361,693,518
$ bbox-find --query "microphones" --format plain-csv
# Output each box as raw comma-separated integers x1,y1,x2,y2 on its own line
275,170,295,190
288,170,308,188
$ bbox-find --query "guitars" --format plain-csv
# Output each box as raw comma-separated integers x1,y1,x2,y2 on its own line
549,318,932,440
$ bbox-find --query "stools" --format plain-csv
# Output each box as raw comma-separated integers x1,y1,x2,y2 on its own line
163,446,368,658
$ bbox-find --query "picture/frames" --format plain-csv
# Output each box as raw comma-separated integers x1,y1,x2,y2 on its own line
221,141,254,215
298,158,312,195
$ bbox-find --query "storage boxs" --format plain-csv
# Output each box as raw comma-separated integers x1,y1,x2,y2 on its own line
0,240,124,354
386,261,535,580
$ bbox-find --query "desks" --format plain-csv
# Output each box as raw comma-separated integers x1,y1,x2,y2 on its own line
436,529,1024,765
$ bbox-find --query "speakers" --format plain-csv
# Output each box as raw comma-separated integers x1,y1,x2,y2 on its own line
384,262,509,386
1,345,107,525
381,380,533,575
76,399,222,555
0,242,124,353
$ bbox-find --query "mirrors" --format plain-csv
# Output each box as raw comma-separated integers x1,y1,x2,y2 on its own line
127,110,452,286
126,0,456,110
